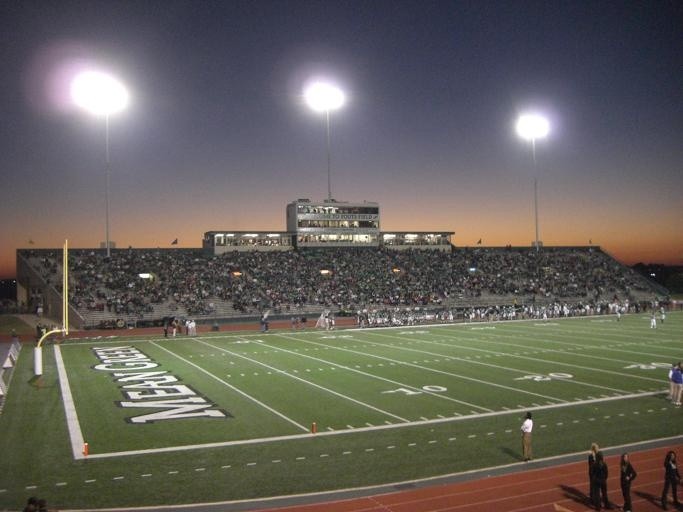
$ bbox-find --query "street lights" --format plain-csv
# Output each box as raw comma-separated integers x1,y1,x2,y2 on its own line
72,72,128,257
515,112,550,248
304,79,343,208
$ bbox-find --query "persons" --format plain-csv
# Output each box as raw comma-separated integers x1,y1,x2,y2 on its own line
671,364,683,405
0,247,683,336
521,412,533,461
665,363,678,400
39,499,49,512
620,453,637,512
21,496,40,512
660,451,683,509
591,451,610,511
589,443,602,505
